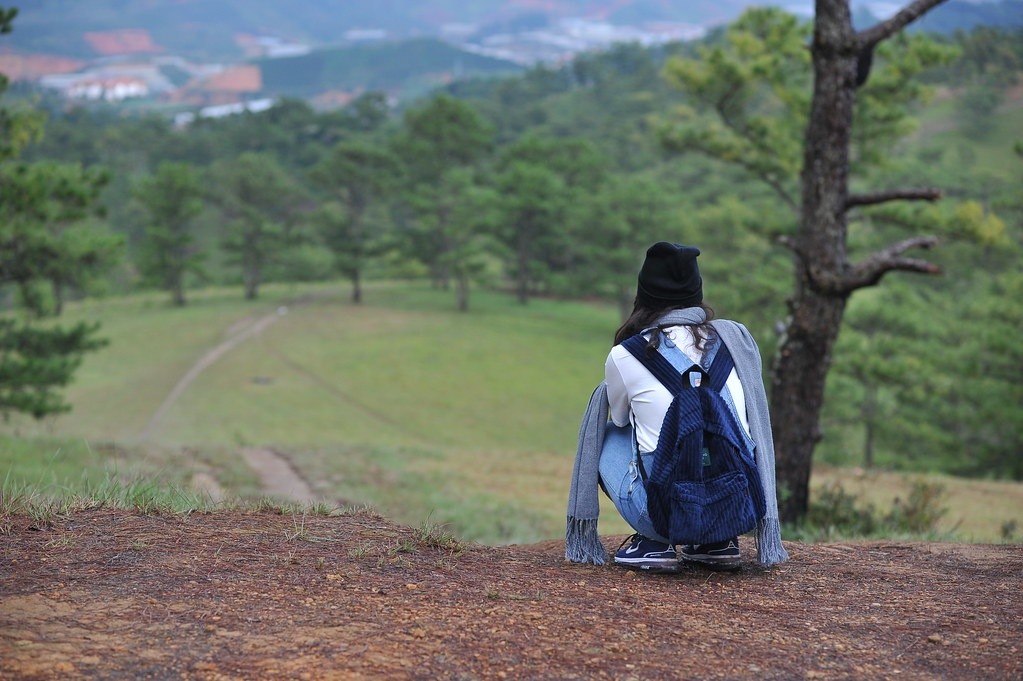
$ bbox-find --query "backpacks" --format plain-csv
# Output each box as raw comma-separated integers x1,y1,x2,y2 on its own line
619,329,767,544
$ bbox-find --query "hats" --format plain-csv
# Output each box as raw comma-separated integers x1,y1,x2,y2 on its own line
636,241,704,308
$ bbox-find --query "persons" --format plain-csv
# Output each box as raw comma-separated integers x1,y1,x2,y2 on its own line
564,242,790,569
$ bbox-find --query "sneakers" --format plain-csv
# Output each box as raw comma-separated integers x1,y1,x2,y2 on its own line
613,533,679,573
678,536,741,569
